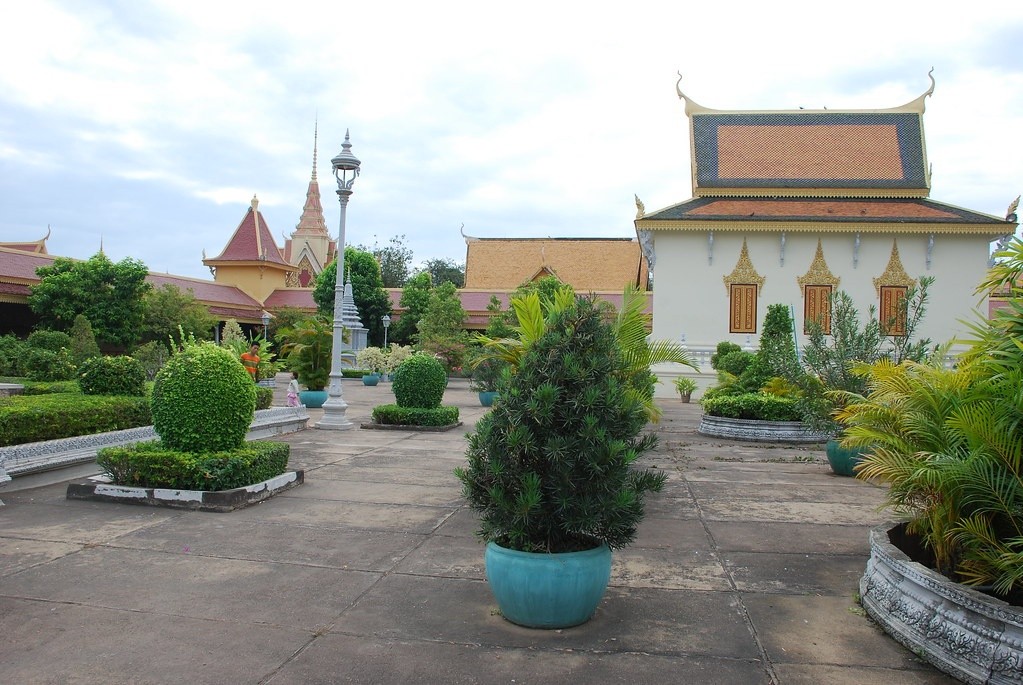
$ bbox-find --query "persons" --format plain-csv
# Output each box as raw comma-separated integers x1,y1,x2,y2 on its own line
240,341,260,383
287,372,300,407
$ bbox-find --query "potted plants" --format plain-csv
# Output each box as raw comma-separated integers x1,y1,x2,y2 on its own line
275,314,356,408
778,275,940,475
673,376,697,404
454,289,668,630
356,346,385,386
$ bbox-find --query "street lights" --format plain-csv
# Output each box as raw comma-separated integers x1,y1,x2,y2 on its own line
314,128,361,430
261,313,270,338
382,315,391,383
